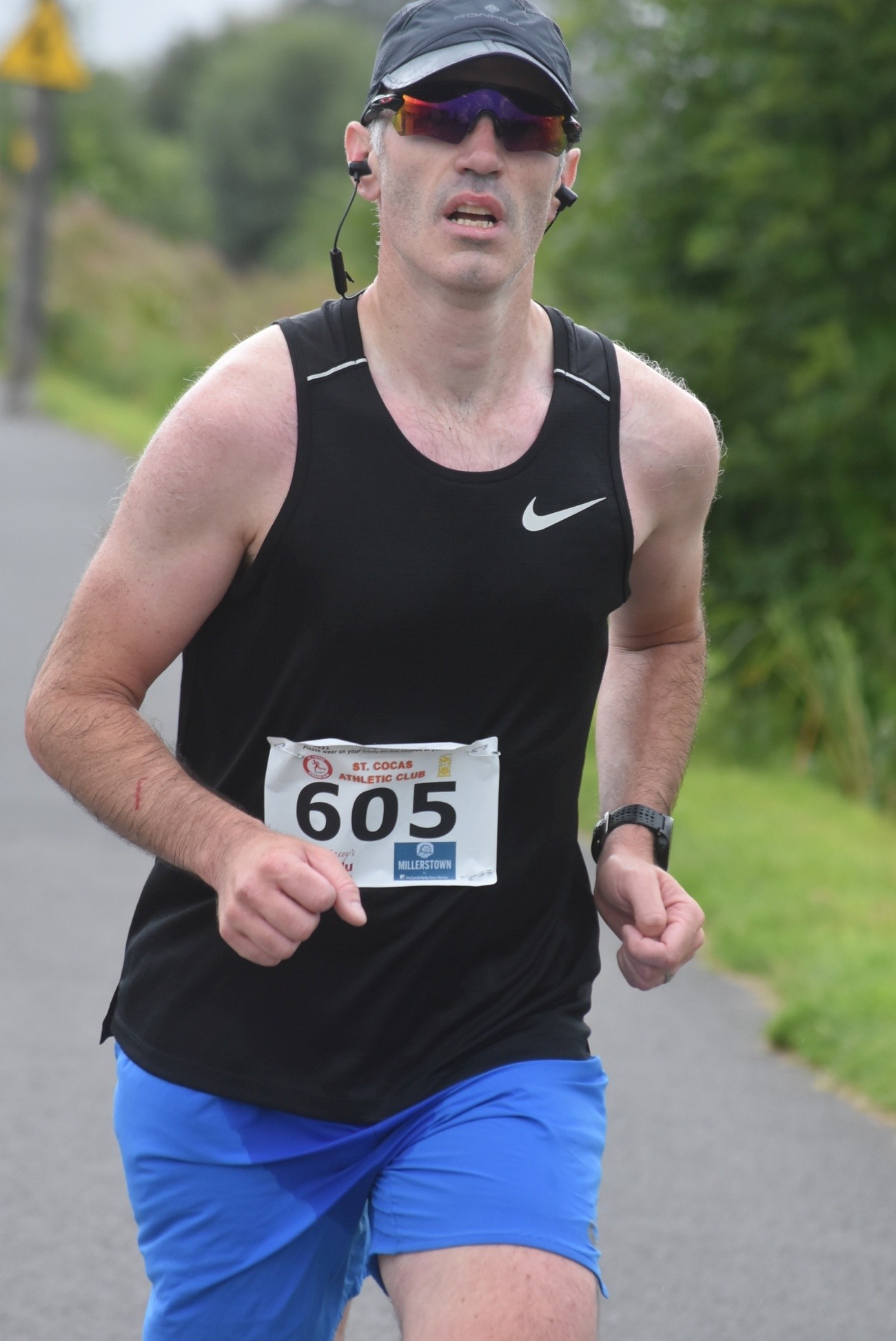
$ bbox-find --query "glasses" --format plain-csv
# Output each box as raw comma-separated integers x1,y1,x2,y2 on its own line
361,82,585,157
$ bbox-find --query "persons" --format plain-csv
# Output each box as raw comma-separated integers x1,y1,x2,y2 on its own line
24,0,721,1341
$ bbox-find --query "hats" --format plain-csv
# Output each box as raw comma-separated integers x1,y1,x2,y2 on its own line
369,0,582,116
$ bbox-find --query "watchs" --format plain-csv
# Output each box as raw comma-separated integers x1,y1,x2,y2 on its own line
591,804,674,871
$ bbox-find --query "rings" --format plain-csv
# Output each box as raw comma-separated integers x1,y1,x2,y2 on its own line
663,970,671,984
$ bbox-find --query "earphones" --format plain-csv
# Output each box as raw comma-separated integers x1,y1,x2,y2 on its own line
349,161,372,181
555,184,578,212
